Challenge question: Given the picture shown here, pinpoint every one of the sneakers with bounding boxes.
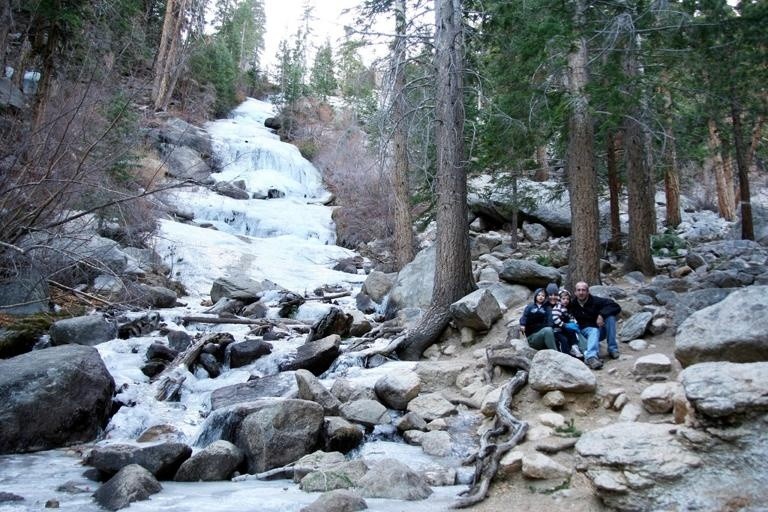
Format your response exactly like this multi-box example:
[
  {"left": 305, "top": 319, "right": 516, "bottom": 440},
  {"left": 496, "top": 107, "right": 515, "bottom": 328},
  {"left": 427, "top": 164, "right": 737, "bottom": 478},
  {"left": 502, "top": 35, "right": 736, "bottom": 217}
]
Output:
[
  {"left": 570, "top": 343, "right": 584, "bottom": 358},
  {"left": 609, "top": 351, "right": 620, "bottom": 359},
  {"left": 587, "top": 356, "right": 604, "bottom": 370}
]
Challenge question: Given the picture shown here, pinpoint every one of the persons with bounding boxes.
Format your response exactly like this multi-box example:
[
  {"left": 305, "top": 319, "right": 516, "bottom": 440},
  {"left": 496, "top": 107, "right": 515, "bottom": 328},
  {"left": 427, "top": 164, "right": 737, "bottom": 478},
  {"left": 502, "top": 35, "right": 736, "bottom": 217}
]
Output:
[{"left": 520, "top": 281, "right": 621, "bottom": 369}]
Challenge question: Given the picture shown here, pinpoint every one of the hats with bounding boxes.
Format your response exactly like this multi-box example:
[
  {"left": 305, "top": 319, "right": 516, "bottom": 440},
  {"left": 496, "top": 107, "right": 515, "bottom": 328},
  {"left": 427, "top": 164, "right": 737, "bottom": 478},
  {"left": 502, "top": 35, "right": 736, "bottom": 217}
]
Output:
[{"left": 546, "top": 283, "right": 559, "bottom": 294}]
